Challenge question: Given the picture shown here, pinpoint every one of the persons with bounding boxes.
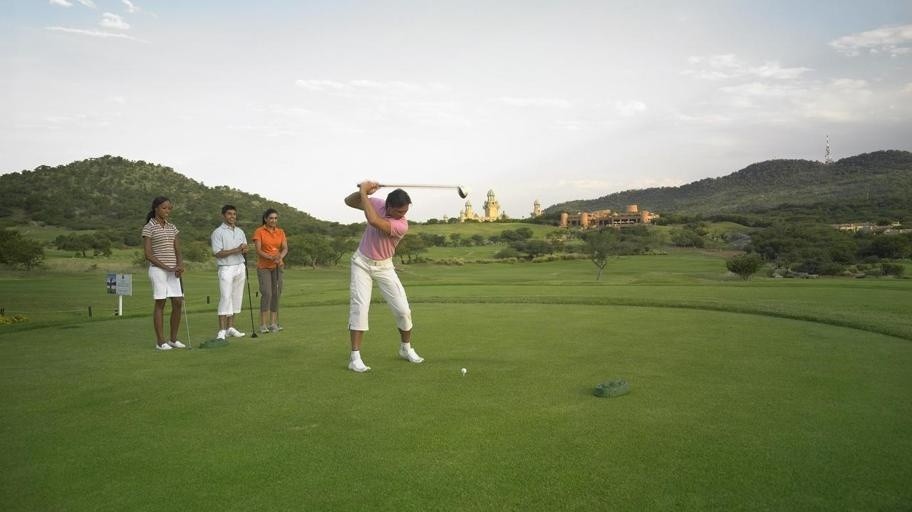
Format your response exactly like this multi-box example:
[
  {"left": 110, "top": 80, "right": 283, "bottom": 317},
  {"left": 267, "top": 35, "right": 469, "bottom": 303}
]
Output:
[
  {"left": 251, "top": 208, "right": 289, "bottom": 333},
  {"left": 140, "top": 196, "right": 186, "bottom": 351},
  {"left": 344, "top": 181, "right": 425, "bottom": 373},
  {"left": 210, "top": 204, "right": 250, "bottom": 341}
]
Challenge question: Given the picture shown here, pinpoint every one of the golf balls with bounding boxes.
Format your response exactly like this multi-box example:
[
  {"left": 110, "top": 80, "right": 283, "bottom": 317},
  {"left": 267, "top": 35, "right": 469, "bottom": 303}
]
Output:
[{"left": 461, "top": 368, "right": 466, "bottom": 374}]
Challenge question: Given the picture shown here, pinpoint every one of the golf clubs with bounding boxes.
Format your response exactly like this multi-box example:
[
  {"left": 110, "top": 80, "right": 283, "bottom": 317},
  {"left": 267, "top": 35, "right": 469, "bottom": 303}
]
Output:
[
  {"left": 180, "top": 274, "right": 192, "bottom": 349},
  {"left": 243, "top": 253, "right": 257, "bottom": 337},
  {"left": 357, "top": 184, "right": 467, "bottom": 199},
  {"left": 276, "top": 263, "right": 283, "bottom": 330}
]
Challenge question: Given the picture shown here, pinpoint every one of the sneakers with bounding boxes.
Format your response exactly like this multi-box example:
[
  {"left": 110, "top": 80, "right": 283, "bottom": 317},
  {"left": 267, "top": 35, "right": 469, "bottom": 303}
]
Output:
[
  {"left": 259, "top": 324, "right": 283, "bottom": 333},
  {"left": 348, "top": 359, "right": 371, "bottom": 373},
  {"left": 156, "top": 340, "right": 186, "bottom": 350},
  {"left": 399, "top": 348, "right": 424, "bottom": 363},
  {"left": 216, "top": 327, "right": 245, "bottom": 340}
]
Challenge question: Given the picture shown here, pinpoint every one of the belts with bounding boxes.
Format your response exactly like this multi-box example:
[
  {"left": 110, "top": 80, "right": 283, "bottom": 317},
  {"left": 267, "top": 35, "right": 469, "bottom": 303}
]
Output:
[{"left": 357, "top": 249, "right": 392, "bottom": 266}]
